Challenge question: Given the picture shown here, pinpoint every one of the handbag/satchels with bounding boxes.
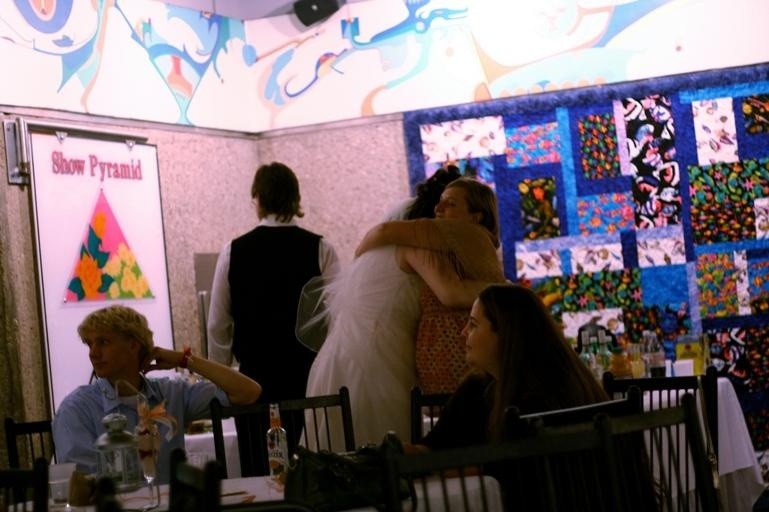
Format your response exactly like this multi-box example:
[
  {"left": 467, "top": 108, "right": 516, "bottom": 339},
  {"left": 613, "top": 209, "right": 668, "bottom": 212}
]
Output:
[{"left": 284, "top": 431, "right": 410, "bottom": 512}]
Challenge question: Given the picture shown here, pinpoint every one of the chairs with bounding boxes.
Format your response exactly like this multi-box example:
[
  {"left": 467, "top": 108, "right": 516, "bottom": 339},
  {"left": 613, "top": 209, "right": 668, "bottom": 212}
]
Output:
[
  {"left": 0, "top": 457, "right": 49, "bottom": 512},
  {"left": 605, "top": 407, "right": 703, "bottom": 512},
  {"left": 706, "top": 366, "right": 769, "bottom": 511},
  {"left": 409, "top": 385, "right": 455, "bottom": 445},
  {"left": 279, "top": 387, "right": 356, "bottom": 462},
  {"left": 209, "top": 397, "right": 271, "bottom": 480},
  {"left": 603, "top": 370, "right": 718, "bottom": 512},
  {"left": 381, "top": 427, "right": 595, "bottom": 512},
  {"left": 3, "top": 417, "right": 59, "bottom": 470}
]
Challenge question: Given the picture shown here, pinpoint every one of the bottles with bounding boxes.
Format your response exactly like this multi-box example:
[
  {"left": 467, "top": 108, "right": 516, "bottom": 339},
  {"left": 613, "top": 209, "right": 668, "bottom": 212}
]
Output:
[
  {"left": 578, "top": 331, "right": 596, "bottom": 369},
  {"left": 649, "top": 331, "right": 666, "bottom": 377},
  {"left": 95, "top": 414, "right": 141, "bottom": 493},
  {"left": 590, "top": 337, "right": 597, "bottom": 358},
  {"left": 267, "top": 403, "right": 290, "bottom": 481},
  {"left": 643, "top": 331, "right": 651, "bottom": 377},
  {"left": 596, "top": 330, "right": 611, "bottom": 369},
  {"left": 611, "top": 348, "right": 634, "bottom": 379}
]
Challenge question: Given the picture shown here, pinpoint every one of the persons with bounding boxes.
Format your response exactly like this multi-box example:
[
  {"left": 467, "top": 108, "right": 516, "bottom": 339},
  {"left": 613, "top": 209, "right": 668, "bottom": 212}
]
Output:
[
  {"left": 52, "top": 306, "right": 263, "bottom": 483},
  {"left": 353, "top": 179, "right": 503, "bottom": 394},
  {"left": 297, "top": 167, "right": 505, "bottom": 451},
  {"left": 206, "top": 162, "right": 339, "bottom": 478},
  {"left": 403, "top": 285, "right": 612, "bottom": 476}
]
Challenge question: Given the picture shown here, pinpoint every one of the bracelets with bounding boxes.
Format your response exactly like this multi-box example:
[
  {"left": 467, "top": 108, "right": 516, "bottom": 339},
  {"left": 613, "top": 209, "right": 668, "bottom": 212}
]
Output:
[{"left": 179, "top": 348, "right": 191, "bottom": 368}]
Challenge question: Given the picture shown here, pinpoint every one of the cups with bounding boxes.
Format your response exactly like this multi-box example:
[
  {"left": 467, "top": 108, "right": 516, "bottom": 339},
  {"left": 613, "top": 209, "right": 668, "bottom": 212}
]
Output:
[{"left": 49, "top": 462, "right": 78, "bottom": 505}]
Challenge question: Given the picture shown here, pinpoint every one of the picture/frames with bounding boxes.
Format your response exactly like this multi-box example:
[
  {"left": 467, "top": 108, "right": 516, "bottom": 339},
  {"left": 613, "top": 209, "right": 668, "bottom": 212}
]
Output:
[{"left": 28, "top": 127, "right": 178, "bottom": 424}]
